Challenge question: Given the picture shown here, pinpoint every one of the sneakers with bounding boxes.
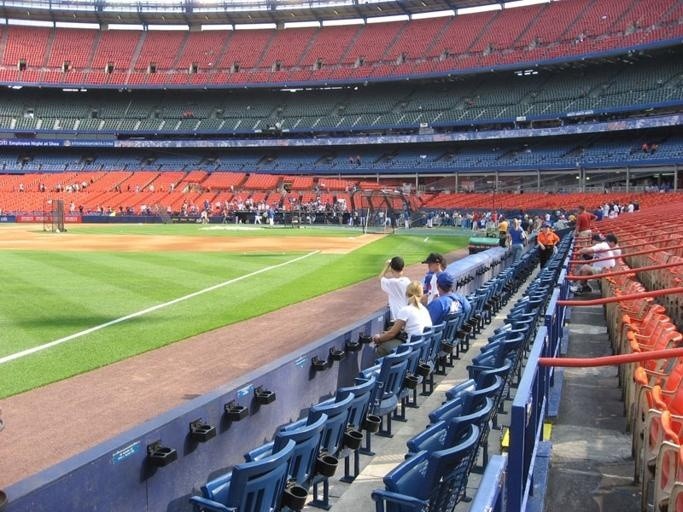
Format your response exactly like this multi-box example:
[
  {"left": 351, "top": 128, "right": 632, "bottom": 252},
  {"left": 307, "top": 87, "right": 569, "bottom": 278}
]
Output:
[{"left": 568, "top": 279, "right": 593, "bottom": 296}]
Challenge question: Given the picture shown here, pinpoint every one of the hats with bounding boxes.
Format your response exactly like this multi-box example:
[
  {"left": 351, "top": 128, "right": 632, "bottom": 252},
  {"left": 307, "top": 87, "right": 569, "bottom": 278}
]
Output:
[
  {"left": 436, "top": 271, "right": 455, "bottom": 289},
  {"left": 420, "top": 252, "right": 444, "bottom": 265},
  {"left": 604, "top": 232, "right": 618, "bottom": 242},
  {"left": 592, "top": 233, "right": 606, "bottom": 242},
  {"left": 389, "top": 256, "right": 404, "bottom": 268},
  {"left": 542, "top": 222, "right": 549, "bottom": 228}
]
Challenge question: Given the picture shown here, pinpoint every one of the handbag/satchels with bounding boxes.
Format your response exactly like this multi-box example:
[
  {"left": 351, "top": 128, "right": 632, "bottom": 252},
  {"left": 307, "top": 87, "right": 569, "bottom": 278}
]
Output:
[{"left": 519, "top": 227, "right": 526, "bottom": 241}]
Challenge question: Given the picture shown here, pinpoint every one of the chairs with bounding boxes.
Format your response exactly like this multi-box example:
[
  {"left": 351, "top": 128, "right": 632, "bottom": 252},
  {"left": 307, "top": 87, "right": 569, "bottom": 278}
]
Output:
[
  {"left": 190, "top": 393, "right": 502, "bottom": 510},
  {"left": 334, "top": 266, "right": 503, "bottom": 402},
  {"left": 502, "top": 2, "right": 681, "bottom": 512},
  {"left": 0, "top": 12, "right": 503, "bottom": 213}
]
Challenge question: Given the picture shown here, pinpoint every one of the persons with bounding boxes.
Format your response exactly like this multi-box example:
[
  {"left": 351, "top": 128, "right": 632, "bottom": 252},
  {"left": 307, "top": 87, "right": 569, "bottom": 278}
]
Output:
[{"left": 640, "top": 143, "right": 659, "bottom": 157}]
